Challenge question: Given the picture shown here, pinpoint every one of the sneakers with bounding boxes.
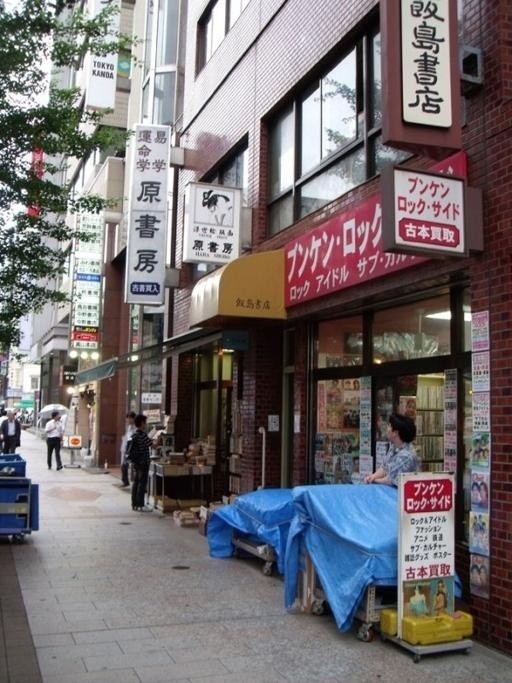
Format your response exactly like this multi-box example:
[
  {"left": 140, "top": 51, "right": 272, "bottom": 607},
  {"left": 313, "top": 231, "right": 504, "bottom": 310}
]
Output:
[{"left": 132, "top": 505, "right": 153, "bottom": 512}]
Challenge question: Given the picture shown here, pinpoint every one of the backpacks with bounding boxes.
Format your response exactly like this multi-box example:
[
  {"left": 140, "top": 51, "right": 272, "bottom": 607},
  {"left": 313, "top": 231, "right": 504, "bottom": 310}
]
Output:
[{"left": 123, "top": 436, "right": 140, "bottom": 463}]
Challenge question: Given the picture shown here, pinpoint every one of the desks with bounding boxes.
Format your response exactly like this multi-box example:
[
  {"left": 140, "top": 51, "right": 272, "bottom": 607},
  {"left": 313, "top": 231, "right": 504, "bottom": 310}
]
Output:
[{"left": 147, "top": 460, "right": 214, "bottom": 513}]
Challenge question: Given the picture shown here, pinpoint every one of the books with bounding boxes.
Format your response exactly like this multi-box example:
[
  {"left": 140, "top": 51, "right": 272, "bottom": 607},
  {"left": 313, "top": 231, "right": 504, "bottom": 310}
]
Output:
[
  {"left": 149, "top": 435, "right": 217, "bottom": 475},
  {"left": 149, "top": 488, "right": 238, "bottom": 528},
  {"left": 415, "top": 385, "right": 444, "bottom": 472}
]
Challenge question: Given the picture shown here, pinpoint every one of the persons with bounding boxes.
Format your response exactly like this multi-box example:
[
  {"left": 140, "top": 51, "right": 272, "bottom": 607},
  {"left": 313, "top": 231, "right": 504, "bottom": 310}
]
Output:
[
  {"left": 44, "top": 411, "right": 64, "bottom": 471},
  {"left": 14, "top": 409, "right": 33, "bottom": 426},
  {"left": 474, "top": 566, "right": 489, "bottom": 594},
  {"left": 325, "top": 378, "right": 362, "bottom": 484},
  {"left": 0, "top": 409, "right": 21, "bottom": 454},
  {"left": 404, "top": 398, "right": 417, "bottom": 418},
  {"left": 434, "top": 580, "right": 449, "bottom": 611},
  {"left": 470, "top": 434, "right": 489, "bottom": 546},
  {"left": 364, "top": 413, "right": 418, "bottom": 486},
  {"left": 470, "top": 564, "right": 479, "bottom": 592},
  {"left": 410, "top": 587, "right": 427, "bottom": 616},
  {"left": 124, "top": 415, "right": 160, "bottom": 512},
  {"left": 120, "top": 411, "right": 136, "bottom": 486},
  {"left": 0, "top": 411, "right": 8, "bottom": 451}
]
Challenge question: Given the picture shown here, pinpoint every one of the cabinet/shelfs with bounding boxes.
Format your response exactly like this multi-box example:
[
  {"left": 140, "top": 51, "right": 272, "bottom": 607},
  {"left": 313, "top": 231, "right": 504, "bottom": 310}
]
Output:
[{"left": 415, "top": 374, "right": 445, "bottom": 474}]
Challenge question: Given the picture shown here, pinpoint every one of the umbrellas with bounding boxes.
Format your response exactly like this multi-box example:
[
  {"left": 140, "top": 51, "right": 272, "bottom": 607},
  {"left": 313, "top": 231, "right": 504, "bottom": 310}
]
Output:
[{"left": 39, "top": 403, "right": 69, "bottom": 413}]
